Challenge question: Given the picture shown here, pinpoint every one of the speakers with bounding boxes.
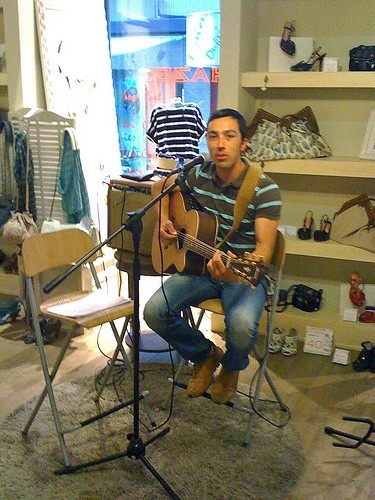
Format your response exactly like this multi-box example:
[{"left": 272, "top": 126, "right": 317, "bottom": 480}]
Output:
[{"left": 106, "top": 177, "right": 158, "bottom": 257}]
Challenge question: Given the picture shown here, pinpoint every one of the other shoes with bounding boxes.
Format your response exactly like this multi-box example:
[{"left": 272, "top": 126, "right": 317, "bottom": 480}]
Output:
[{"left": 352, "top": 341, "right": 375, "bottom": 373}]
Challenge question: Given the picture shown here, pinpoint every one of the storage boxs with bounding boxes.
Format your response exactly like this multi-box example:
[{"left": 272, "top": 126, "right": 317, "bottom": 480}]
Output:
[{"left": 107, "top": 177, "right": 157, "bottom": 256}]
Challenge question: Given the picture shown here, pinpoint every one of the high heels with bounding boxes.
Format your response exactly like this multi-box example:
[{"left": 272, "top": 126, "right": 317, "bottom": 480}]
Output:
[
  {"left": 298, "top": 211, "right": 314, "bottom": 240},
  {"left": 0, "top": 297, "right": 21, "bottom": 325},
  {"left": 268, "top": 327, "right": 299, "bottom": 356},
  {"left": 290, "top": 47, "right": 327, "bottom": 72},
  {"left": 348, "top": 271, "right": 365, "bottom": 306},
  {"left": 314, "top": 215, "right": 332, "bottom": 242},
  {"left": 281, "top": 18, "right": 295, "bottom": 56},
  {"left": 359, "top": 311, "right": 375, "bottom": 323},
  {"left": 24, "top": 317, "right": 61, "bottom": 346}
]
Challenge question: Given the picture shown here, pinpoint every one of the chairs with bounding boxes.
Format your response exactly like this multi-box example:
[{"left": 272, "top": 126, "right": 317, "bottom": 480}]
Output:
[
  {"left": 21, "top": 228, "right": 156, "bottom": 468},
  {"left": 161, "top": 226, "right": 286, "bottom": 446}
]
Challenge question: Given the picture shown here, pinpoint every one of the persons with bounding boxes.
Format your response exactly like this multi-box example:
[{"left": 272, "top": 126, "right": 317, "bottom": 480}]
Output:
[{"left": 143, "top": 109, "right": 281, "bottom": 405}]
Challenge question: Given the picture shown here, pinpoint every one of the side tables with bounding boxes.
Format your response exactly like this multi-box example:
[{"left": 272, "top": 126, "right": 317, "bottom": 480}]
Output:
[{"left": 113, "top": 251, "right": 188, "bottom": 352}]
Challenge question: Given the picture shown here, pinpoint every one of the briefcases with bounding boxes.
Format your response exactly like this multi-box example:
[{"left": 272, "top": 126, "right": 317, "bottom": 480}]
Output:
[{"left": 108, "top": 169, "right": 172, "bottom": 256}]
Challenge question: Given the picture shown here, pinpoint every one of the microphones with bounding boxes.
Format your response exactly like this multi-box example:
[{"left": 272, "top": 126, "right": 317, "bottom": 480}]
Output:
[{"left": 174, "top": 154, "right": 206, "bottom": 174}]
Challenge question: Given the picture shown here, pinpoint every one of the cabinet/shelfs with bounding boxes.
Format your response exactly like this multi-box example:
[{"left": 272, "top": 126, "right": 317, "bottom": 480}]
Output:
[{"left": 212, "top": 0, "right": 375, "bottom": 350}]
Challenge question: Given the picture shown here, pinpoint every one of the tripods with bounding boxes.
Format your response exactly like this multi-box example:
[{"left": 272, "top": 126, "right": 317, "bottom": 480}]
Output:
[{"left": 42, "top": 169, "right": 189, "bottom": 500}]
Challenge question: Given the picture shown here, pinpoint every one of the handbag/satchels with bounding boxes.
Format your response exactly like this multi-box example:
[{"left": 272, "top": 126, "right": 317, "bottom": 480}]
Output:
[
  {"left": 244, "top": 106, "right": 331, "bottom": 162},
  {"left": 265, "top": 285, "right": 323, "bottom": 313},
  {"left": 329, "top": 194, "right": 375, "bottom": 254},
  {"left": 3, "top": 213, "right": 38, "bottom": 244},
  {"left": 349, "top": 45, "right": 375, "bottom": 71}
]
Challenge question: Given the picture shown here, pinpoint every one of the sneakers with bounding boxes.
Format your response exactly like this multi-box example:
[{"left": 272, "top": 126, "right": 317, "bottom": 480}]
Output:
[
  {"left": 187, "top": 341, "right": 223, "bottom": 397},
  {"left": 211, "top": 366, "right": 238, "bottom": 405}
]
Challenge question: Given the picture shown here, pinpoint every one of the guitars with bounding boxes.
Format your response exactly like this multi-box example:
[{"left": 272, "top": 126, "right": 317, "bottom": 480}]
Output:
[{"left": 151, "top": 191, "right": 269, "bottom": 290}]
